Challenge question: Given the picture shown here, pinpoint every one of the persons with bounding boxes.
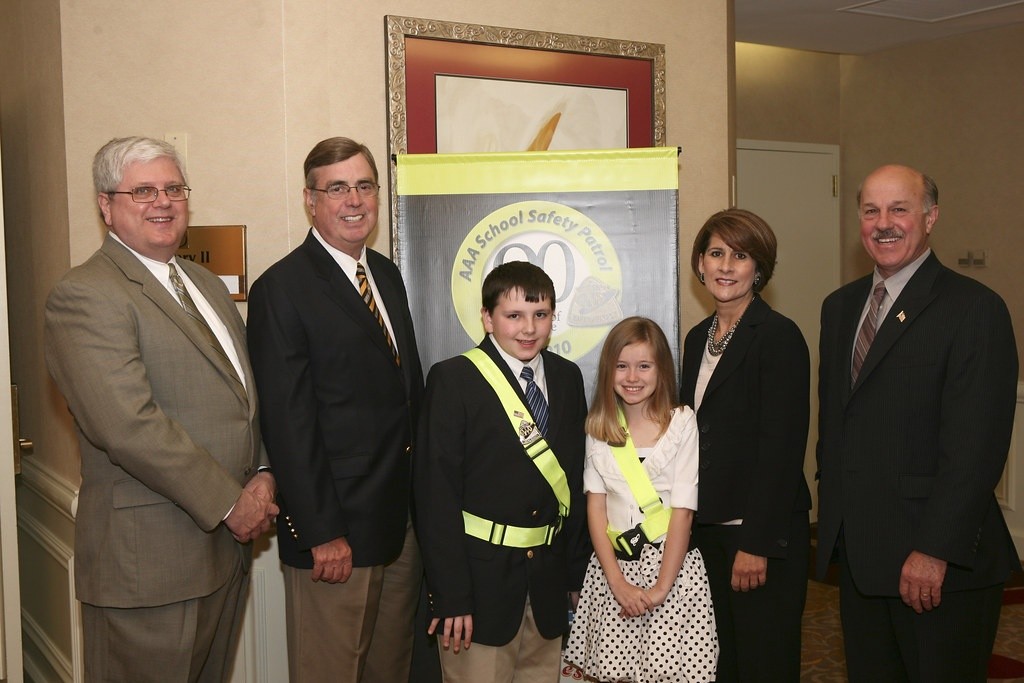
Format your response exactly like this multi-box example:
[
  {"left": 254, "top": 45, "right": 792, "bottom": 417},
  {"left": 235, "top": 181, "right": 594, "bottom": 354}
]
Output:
[
  {"left": 817, "top": 163, "right": 1022, "bottom": 683},
  {"left": 248, "top": 138, "right": 424, "bottom": 683},
  {"left": 680, "top": 206, "right": 814, "bottom": 576},
  {"left": 415, "top": 259, "right": 589, "bottom": 683},
  {"left": 564, "top": 316, "right": 722, "bottom": 683},
  {"left": 44, "top": 133, "right": 277, "bottom": 683}
]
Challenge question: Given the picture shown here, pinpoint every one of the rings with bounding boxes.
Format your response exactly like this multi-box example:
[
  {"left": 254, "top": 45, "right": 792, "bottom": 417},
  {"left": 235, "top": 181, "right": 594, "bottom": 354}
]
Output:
[{"left": 921, "top": 594, "right": 930, "bottom": 601}]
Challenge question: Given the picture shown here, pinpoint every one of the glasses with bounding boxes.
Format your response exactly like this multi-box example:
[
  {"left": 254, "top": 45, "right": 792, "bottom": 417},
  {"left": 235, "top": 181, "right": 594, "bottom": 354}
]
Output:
[
  {"left": 108, "top": 184, "right": 192, "bottom": 203},
  {"left": 310, "top": 183, "right": 380, "bottom": 200}
]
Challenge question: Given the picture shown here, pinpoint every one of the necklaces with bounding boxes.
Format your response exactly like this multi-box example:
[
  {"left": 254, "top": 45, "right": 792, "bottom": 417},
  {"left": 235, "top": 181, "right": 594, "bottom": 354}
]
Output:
[{"left": 710, "top": 310, "right": 745, "bottom": 355}]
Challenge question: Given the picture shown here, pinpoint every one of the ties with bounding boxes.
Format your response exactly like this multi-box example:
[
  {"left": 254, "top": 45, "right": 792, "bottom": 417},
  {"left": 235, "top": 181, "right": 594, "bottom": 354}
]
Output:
[
  {"left": 356, "top": 263, "right": 401, "bottom": 370},
  {"left": 167, "top": 263, "right": 249, "bottom": 405},
  {"left": 520, "top": 367, "right": 549, "bottom": 439},
  {"left": 850, "top": 281, "right": 887, "bottom": 391}
]
